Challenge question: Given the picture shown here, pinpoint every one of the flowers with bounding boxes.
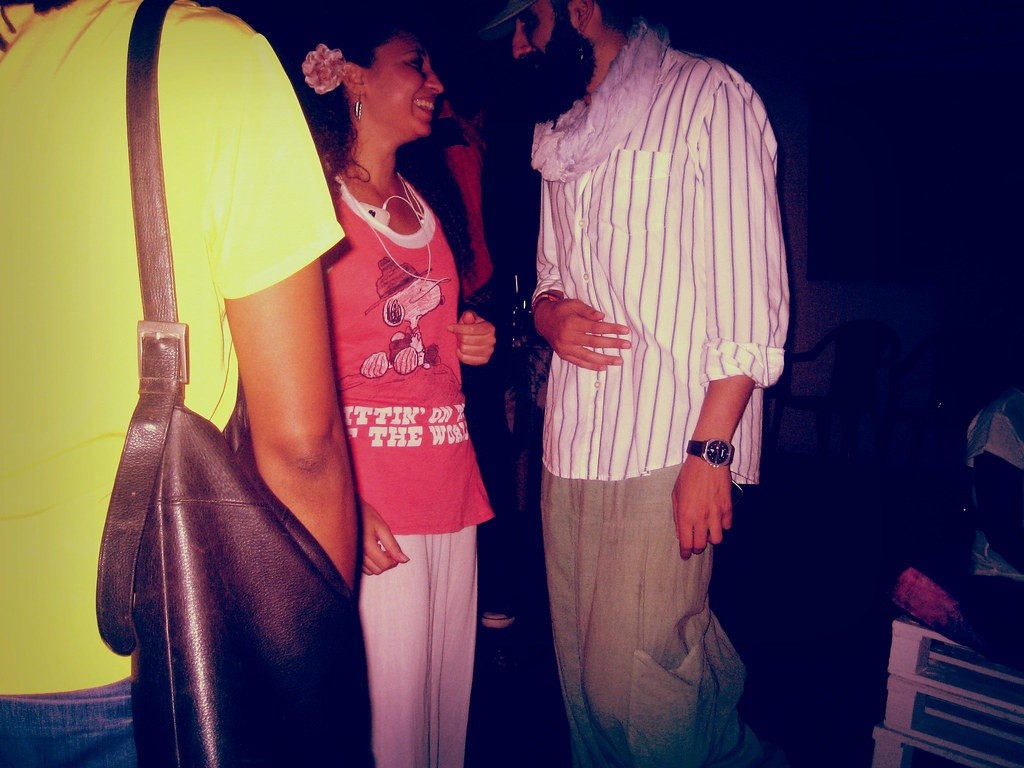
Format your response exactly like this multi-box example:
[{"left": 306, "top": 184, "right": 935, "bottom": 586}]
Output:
[{"left": 300, "top": 44, "right": 345, "bottom": 95}]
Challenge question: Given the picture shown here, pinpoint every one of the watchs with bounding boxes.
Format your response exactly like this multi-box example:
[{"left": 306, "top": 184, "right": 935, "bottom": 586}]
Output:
[{"left": 685, "top": 437, "right": 735, "bottom": 468}]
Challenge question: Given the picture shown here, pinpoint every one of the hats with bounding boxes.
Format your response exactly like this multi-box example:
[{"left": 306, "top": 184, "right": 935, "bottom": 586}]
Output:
[{"left": 478, "top": 0, "right": 539, "bottom": 41}]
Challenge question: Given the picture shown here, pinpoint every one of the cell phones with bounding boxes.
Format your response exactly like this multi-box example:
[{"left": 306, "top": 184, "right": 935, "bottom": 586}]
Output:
[{"left": 357, "top": 202, "right": 390, "bottom": 228}]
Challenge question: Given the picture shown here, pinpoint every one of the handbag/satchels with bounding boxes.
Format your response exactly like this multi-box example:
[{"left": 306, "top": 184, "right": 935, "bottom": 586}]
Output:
[{"left": 97, "top": 318, "right": 370, "bottom": 768}]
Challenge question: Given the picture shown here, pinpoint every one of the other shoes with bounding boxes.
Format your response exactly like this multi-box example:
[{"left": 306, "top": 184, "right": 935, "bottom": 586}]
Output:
[{"left": 482, "top": 574, "right": 523, "bottom": 628}]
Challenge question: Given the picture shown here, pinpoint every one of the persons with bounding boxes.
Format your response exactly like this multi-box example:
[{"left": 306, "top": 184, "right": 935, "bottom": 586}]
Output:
[
  {"left": 483, "top": 0, "right": 790, "bottom": 768},
  {"left": 0, "top": 0, "right": 497, "bottom": 768},
  {"left": 965, "top": 384, "right": 1024, "bottom": 655}
]
